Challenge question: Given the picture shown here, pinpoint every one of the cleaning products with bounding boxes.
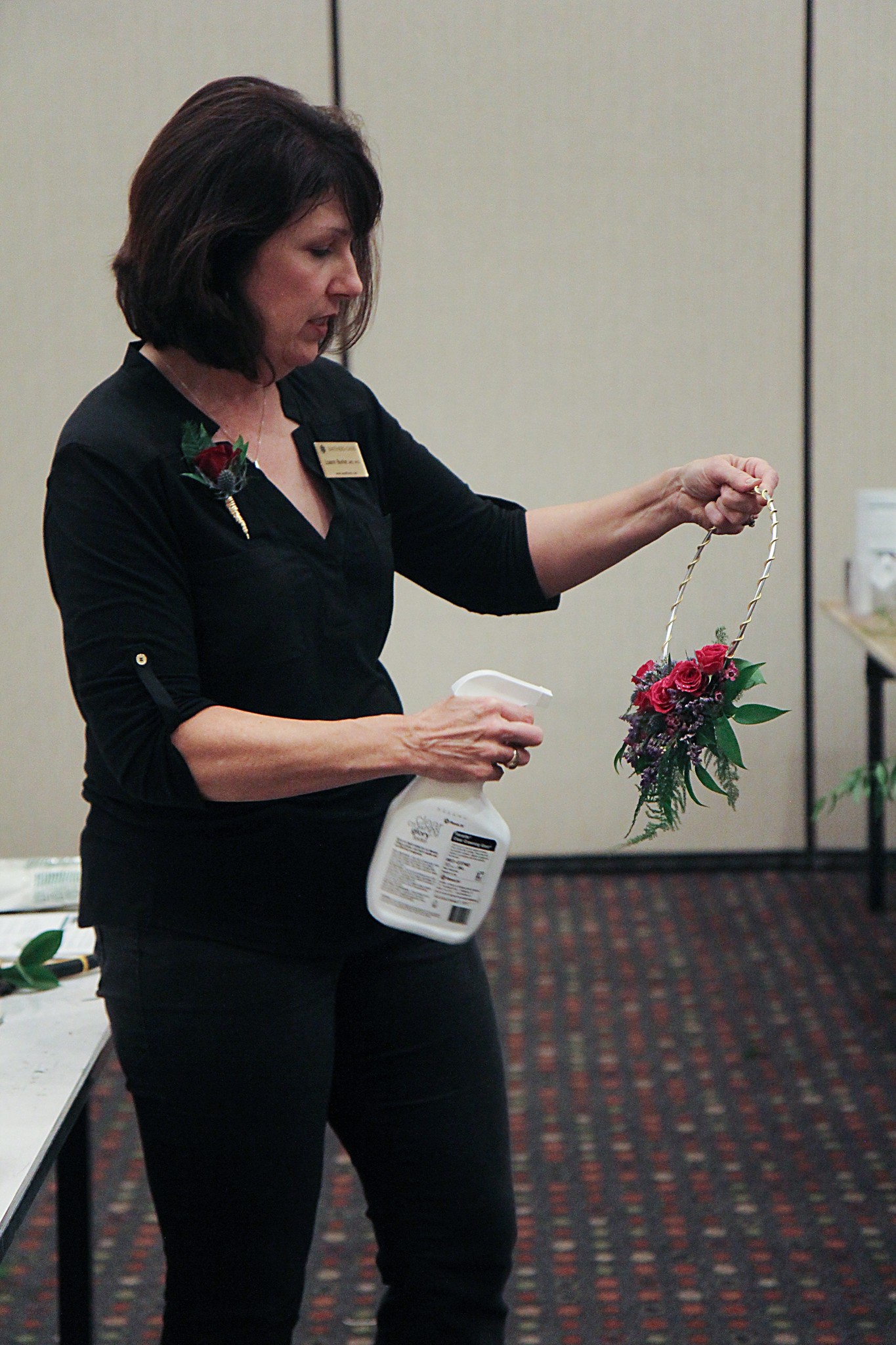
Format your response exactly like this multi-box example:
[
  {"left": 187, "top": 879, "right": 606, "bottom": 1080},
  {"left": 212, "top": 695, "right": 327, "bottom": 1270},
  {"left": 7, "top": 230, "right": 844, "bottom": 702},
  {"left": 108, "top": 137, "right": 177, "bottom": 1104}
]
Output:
[{"left": 367, "top": 671, "right": 553, "bottom": 946}]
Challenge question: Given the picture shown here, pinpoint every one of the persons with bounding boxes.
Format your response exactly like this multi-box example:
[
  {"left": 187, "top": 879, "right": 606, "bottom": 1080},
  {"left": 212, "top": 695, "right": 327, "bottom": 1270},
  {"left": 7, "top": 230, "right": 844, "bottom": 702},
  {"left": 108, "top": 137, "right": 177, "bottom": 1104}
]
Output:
[{"left": 42, "top": 74, "right": 781, "bottom": 1345}]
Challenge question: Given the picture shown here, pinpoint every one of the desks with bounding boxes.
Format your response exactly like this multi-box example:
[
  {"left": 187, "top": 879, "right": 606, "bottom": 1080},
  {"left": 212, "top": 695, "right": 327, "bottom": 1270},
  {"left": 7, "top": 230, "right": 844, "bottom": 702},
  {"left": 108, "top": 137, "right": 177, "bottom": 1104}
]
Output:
[
  {"left": 815, "top": 597, "right": 896, "bottom": 916},
  {"left": 0, "top": 856, "right": 116, "bottom": 1345}
]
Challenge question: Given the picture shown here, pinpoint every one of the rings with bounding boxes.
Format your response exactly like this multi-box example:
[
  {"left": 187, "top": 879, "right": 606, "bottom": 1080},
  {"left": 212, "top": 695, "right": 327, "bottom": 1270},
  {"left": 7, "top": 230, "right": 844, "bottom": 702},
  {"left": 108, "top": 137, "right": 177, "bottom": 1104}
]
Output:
[{"left": 506, "top": 749, "right": 519, "bottom": 769}]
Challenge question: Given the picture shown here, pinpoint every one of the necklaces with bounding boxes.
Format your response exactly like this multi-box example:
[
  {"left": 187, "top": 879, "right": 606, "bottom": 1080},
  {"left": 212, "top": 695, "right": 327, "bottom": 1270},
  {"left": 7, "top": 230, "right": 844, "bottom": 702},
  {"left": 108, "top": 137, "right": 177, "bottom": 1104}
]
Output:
[{"left": 151, "top": 348, "right": 266, "bottom": 470}]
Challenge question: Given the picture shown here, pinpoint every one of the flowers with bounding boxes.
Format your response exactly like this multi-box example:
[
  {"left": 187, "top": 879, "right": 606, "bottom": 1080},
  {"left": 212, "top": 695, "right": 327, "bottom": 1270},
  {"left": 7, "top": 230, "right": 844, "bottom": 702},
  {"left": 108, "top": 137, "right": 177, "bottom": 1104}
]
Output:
[{"left": 611, "top": 613, "right": 789, "bottom": 850}]
